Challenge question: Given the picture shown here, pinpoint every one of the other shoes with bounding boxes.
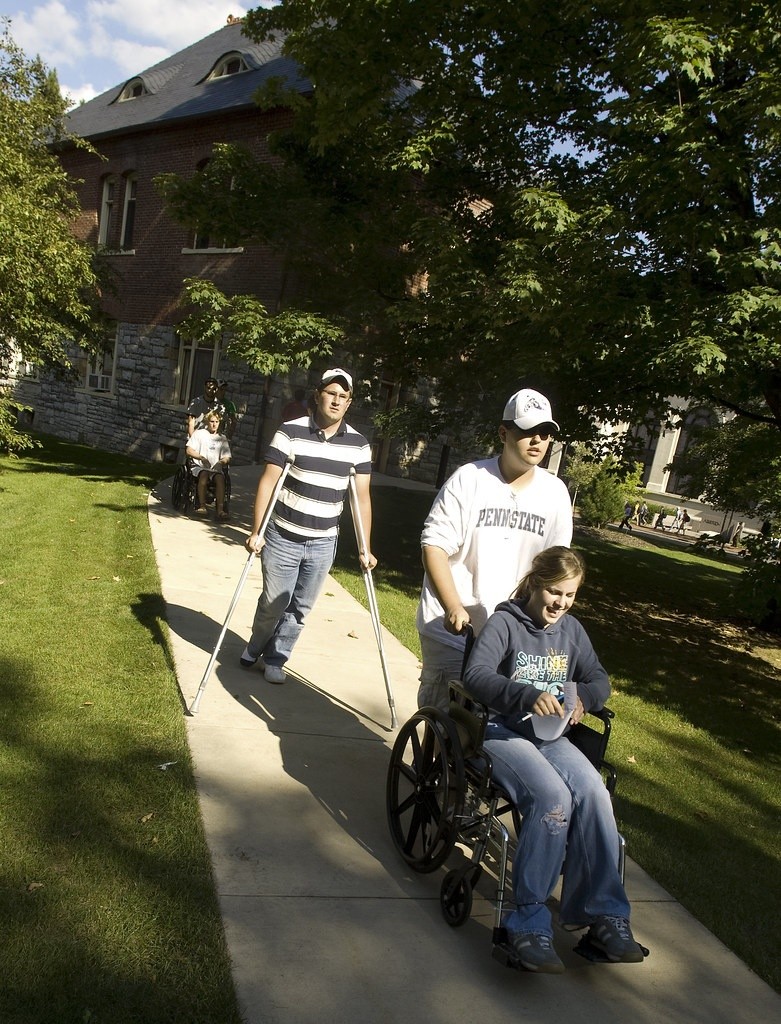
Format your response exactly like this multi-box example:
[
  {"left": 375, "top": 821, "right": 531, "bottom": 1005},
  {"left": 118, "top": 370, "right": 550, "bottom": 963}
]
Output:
[
  {"left": 197, "top": 506, "right": 207, "bottom": 515},
  {"left": 217, "top": 509, "right": 229, "bottom": 519},
  {"left": 439, "top": 801, "right": 482, "bottom": 843}
]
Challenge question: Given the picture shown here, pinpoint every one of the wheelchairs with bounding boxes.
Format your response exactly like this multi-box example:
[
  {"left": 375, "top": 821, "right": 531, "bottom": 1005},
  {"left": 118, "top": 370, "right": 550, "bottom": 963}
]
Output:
[
  {"left": 171, "top": 439, "right": 231, "bottom": 520},
  {"left": 384, "top": 621, "right": 651, "bottom": 972}
]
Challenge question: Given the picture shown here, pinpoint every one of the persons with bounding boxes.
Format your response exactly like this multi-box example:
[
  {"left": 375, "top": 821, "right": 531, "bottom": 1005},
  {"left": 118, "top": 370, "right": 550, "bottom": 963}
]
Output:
[
  {"left": 183, "top": 377, "right": 237, "bottom": 521},
  {"left": 618, "top": 499, "right": 689, "bottom": 537},
  {"left": 730, "top": 522, "right": 746, "bottom": 548},
  {"left": 461, "top": 544, "right": 646, "bottom": 975},
  {"left": 280, "top": 390, "right": 309, "bottom": 425},
  {"left": 412, "top": 390, "right": 574, "bottom": 756},
  {"left": 239, "top": 367, "right": 379, "bottom": 682}
]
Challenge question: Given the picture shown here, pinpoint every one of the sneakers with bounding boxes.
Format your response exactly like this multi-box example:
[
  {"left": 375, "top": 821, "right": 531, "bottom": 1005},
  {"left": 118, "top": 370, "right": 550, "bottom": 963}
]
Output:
[
  {"left": 507, "top": 928, "right": 563, "bottom": 974},
  {"left": 589, "top": 914, "right": 643, "bottom": 963},
  {"left": 264, "top": 656, "right": 286, "bottom": 684}
]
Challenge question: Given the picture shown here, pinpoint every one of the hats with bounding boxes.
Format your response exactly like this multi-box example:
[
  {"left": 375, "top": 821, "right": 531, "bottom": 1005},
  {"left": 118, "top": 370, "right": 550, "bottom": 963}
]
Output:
[
  {"left": 216, "top": 378, "right": 228, "bottom": 388},
  {"left": 319, "top": 370, "right": 354, "bottom": 394},
  {"left": 503, "top": 389, "right": 561, "bottom": 434}
]
